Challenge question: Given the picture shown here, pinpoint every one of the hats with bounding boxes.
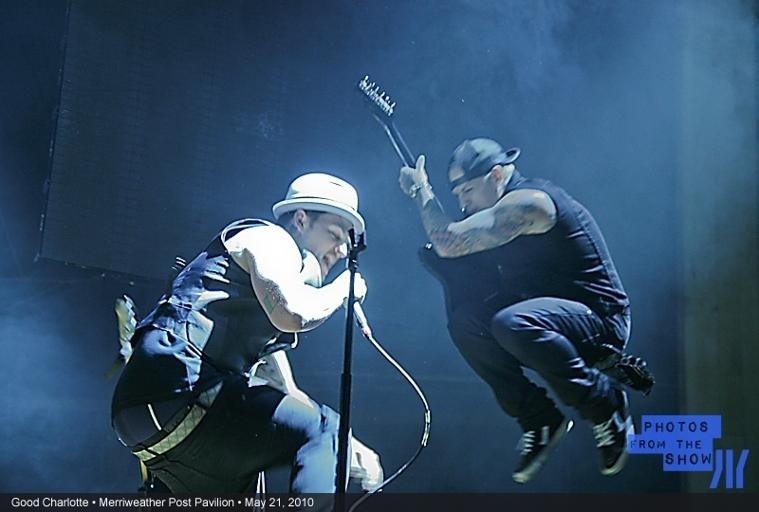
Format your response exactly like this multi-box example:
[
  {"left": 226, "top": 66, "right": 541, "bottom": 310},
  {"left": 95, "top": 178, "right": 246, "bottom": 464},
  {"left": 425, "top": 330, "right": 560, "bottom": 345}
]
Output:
[
  {"left": 272, "top": 172, "right": 366, "bottom": 239},
  {"left": 446, "top": 137, "right": 521, "bottom": 193}
]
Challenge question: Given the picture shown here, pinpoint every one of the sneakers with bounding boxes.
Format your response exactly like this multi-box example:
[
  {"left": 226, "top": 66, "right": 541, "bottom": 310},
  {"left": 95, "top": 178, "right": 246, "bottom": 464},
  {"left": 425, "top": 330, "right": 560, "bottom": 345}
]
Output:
[
  {"left": 511, "top": 406, "right": 575, "bottom": 485},
  {"left": 590, "top": 389, "right": 637, "bottom": 476}
]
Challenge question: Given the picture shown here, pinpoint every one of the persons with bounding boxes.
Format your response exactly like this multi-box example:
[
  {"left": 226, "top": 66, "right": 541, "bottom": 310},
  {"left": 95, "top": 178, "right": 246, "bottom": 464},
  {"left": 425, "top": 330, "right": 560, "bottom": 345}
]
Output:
[
  {"left": 396, "top": 137, "right": 637, "bottom": 484},
  {"left": 107, "top": 173, "right": 386, "bottom": 501}
]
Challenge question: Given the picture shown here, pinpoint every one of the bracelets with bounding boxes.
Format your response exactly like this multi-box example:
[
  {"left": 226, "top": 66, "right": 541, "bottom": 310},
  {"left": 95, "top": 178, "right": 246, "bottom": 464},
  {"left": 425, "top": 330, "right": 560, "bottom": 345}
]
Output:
[{"left": 407, "top": 181, "right": 434, "bottom": 203}]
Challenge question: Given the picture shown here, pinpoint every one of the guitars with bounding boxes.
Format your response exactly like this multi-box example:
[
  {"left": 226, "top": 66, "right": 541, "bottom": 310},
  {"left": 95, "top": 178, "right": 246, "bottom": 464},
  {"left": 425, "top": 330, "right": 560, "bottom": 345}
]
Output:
[{"left": 357, "top": 75, "right": 503, "bottom": 321}]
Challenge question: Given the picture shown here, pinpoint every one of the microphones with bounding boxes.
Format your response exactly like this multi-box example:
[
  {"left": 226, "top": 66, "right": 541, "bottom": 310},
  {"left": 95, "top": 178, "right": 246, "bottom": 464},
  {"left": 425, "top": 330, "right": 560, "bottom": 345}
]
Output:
[{"left": 339, "top": 290, "right": 374, "bottom": 339}]
